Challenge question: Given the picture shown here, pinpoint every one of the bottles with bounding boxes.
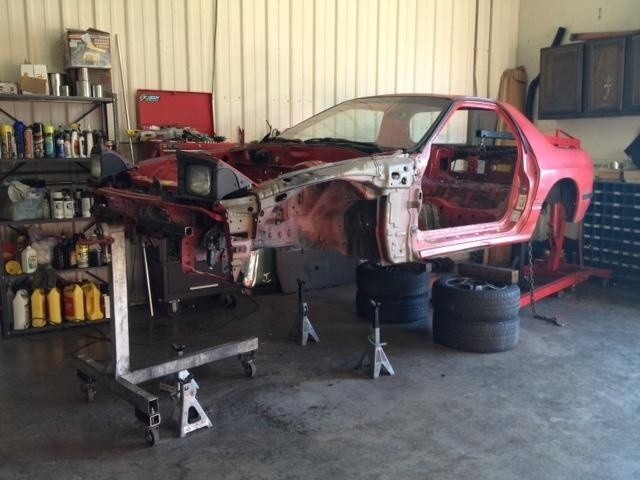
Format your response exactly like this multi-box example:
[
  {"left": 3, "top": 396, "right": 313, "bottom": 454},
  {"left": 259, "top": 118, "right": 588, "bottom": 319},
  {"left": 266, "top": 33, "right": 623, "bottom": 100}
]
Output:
[
  {"left": 22, "top": 242, "right": 37, "bottom": 273},
  {"left": 58, "top": 133, "right": 65, "bottom": 158},
  {"left": 44, "top": 125, "right": 56, "bottom": 157},
  {"left": 34, "top": 123, "right": 45, "bottom": 158},
  {"left": 25, "top": 128, "right": 34, "bottom": 158},
  {"left": 65, "top": 131, "right": 71, "bottom": 158},
  {"left": 75, "top": 188, "right": 82, "bottom": 216},
  {"left": 82, "top": 190, "right": 92, "bottom": 217},
  {"left": 76, "top": 239, "right": 89, "bottom": 268},
  {"left": 2, "top": 124, "right": 12, "bottom": 159},
  {"left": 90, "top": 233, "right": 102, "bottom": 267},
  {"left": 64, "top": 194, "right": 73, "bottom": 218},
  {"left": 52, "top": 192, "right": 64, "bottom": 219},
  {"left": 14, "top": 121, "right": 25, "bottom": 159}
]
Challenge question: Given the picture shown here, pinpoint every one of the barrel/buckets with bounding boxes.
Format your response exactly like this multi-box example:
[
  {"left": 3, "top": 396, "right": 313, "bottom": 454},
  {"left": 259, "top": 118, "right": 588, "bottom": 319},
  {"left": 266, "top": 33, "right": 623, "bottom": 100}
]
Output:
[
  {"left": 31, "top": 288, "right": 47, "bottom": 327},
  {"left": 64, "top": 284, "right": 84, "bottom": 322},
  {"left": 81, "top": 283, "right": 104, "bottom": 320},
  {"left": 47, "top": 287, "right": 62, "bottom": 325},
  {"left": 13, "top": 289, "right": 30, "bottom": 330}
]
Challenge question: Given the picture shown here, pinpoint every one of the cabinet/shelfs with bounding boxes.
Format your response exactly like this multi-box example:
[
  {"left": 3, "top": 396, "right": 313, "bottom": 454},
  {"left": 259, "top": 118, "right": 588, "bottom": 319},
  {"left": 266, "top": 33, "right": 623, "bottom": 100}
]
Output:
[
  {"left": 629, "top": 35, "right": 640, "bottom": 110},
  {"left": 539, "top": 43, "right": 583, "bottom": 115},
  {"left": 0, "top": 90, "right": 120, "bottom": 341},
  {"left": 586, "top": 38, "right": 623, "bottom": 112}
]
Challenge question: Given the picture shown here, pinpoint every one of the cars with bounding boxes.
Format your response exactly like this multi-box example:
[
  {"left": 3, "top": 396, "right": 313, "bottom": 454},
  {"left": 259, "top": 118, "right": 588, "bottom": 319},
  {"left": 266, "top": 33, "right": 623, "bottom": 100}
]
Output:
[{"left": 86, "top": 92, "right": 595, "bottom": 290}]
